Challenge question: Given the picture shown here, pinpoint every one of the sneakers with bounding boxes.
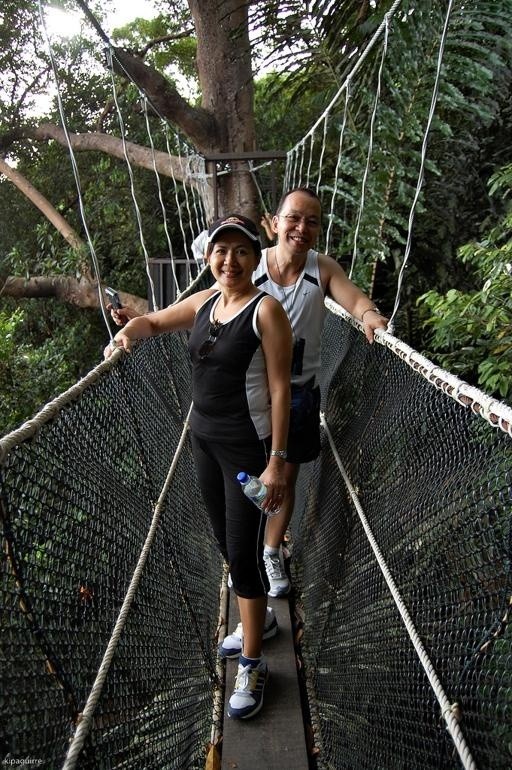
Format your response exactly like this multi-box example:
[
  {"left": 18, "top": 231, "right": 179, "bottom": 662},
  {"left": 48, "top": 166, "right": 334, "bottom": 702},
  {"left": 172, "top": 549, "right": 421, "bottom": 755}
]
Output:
[
  {"left": 228, "top": 662, "right": 268, "bottom": 720},
  {"left": 216, "top": 609, "right": 276, "bottom": 659},
  {"left": 263, "top": 553, "right": 292, "bottom": 599}
]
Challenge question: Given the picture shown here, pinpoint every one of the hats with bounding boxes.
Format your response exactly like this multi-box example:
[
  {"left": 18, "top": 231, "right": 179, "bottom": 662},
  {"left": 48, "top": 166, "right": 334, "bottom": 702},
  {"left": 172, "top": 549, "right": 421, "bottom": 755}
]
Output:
[{"left": 208, "top": 215, "right": 263, "bottom": 247}]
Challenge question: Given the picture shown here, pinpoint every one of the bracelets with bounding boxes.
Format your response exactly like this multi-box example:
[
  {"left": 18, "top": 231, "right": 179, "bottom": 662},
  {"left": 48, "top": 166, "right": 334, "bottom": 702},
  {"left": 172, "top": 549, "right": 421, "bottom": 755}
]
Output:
[{"left": 359, "top": 307, "right": 384, "bottom": 322}]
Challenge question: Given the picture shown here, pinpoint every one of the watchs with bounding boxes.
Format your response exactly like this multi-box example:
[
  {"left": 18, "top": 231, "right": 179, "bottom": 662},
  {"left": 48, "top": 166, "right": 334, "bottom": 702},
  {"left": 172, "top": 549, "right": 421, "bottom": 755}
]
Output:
[{"left": 270, "top": 448, "right": 289, "bottom": 462}]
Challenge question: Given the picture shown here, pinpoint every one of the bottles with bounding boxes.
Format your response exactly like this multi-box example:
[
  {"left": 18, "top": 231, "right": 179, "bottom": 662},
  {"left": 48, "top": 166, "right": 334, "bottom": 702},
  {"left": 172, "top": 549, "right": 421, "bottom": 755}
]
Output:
[{"left": 237, "top": 471, "right": 285, "bottom": 517}]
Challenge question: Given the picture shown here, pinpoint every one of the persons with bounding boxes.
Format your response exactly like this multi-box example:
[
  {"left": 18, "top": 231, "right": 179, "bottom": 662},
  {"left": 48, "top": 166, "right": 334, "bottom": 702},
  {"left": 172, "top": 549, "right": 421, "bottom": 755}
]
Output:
[
  {"left": 103, "top": 186, "right": 391, "bottom": 606},
  {"left": 99, "top": 213, "right": 296, "bottom": 727},
  {"left": 190, "top": 215, "right": 219, "bottom": 272},
  {"left": 258, "top": 210, "right": 279, "bottom": 249}
]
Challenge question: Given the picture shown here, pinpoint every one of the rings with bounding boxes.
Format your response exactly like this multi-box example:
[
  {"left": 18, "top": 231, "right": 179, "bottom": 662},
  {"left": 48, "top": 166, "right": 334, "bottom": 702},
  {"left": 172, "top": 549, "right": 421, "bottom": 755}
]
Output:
[{"left": 277, "top": 493, "right": 283, "bottom": 498}]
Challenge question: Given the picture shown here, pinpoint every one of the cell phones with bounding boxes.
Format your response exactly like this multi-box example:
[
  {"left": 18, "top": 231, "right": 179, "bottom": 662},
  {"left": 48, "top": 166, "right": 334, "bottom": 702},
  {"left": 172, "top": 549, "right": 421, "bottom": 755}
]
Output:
[{"left": 105, "top": 286, "right": 126, "bottom": 325}]
